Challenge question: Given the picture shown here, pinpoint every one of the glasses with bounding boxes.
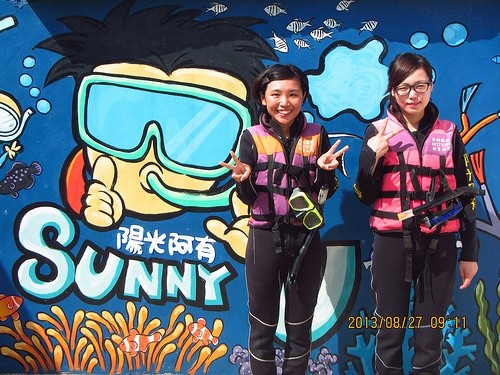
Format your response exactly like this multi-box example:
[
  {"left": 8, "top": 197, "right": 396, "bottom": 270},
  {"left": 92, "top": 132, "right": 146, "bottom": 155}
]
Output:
[
  {"left": 392, "top": 79, "right": 432, "bottom": 96},
  {"left": 290, "top": 189, "right": 323, "bottom": 230}
]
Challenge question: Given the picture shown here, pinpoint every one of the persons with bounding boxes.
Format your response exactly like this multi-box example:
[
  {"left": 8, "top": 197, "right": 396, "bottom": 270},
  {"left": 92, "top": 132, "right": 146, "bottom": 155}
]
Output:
[
  {"left": 219, "top": 62, "right": 349, "bottom": 374},
  {"left": 353, "top": 51, "right": 479, "bottom": 374}
]
